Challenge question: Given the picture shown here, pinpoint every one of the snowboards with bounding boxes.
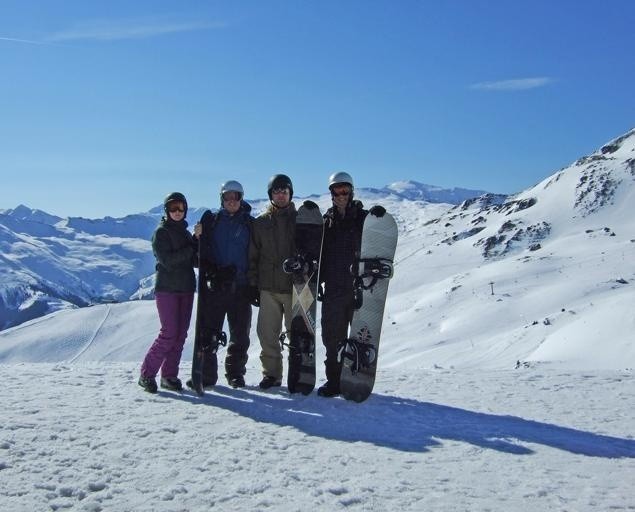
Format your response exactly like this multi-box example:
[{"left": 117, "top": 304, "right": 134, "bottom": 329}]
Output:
[
  {"left": 340, "top": 213, "right": 398, "bottom": 403},
  {"left": 288, "top": 205, "right": 325, "bottom": 396},
  {"left": 192, "top": 210, "right": 211, "bottom": 396}
]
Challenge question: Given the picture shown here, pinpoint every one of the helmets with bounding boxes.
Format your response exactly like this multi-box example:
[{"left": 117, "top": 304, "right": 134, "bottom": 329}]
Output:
[
  {"left": 219, "top": 180, "right": 244, "bottom": 196},
  {"left": 328, "top": 171, "right": 353, "bottom": 189},
  {"left": 164, "top": 192, "right": 187, "bottom": 206},
  {"left": 267, "top": 174, "right": 293, "bottom": 191}
]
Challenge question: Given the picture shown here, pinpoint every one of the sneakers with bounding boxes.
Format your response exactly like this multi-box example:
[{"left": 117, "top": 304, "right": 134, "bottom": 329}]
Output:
[{"left": 138, "top": 371, "right": 340, "bottom": 398}]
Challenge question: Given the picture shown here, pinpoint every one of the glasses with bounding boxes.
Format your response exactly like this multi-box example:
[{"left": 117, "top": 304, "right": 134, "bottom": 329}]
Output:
[
  {"left": 222, "top": 192, "right": 242, "bottom": 201},
  {"left": 166, "top": 201, "right": 187, "bottom": 212},
  {"left": 331, "top": 186, "right": 352, "bottom": 196},
  {"left": 271, "top": 185, "right": 292, "bottom": 194}
]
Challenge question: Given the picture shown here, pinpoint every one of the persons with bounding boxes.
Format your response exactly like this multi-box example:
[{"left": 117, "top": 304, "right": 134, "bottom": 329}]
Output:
[
  {"left": 315, "top": 170, "right": 387, "bottom": 398},
  {"left": 136, "top": 191, "right": 199, "bottom": 395},
  {"left": 245, "top": 173, "right": 320, "bottom": 389},
  {"left": 186, "top": 180, "right": 258, "bottom": 389}
]
